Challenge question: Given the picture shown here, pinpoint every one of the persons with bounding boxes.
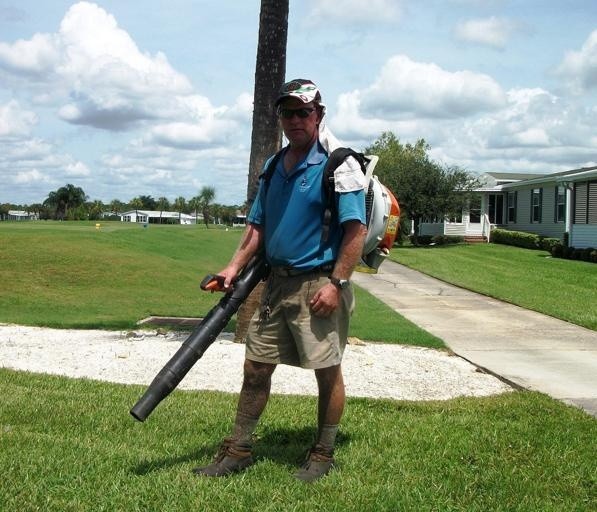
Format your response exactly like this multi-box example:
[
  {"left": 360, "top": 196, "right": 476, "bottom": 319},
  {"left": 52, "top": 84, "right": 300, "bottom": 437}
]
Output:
[{"left": 192, "top": 78, "right": 371, "bottom": 486}]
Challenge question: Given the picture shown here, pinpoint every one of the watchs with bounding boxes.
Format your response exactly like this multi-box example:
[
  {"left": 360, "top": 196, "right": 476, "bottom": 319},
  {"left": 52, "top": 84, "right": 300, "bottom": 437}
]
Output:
[{"left": 331, "top": 276, "right": 348, "bottom": 290}]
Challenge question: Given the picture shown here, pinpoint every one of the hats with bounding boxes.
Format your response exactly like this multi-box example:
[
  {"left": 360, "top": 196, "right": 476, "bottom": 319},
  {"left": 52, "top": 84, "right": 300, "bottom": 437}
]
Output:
[{"left": 274, "top": 79, "right": 326, "bottom": 108}]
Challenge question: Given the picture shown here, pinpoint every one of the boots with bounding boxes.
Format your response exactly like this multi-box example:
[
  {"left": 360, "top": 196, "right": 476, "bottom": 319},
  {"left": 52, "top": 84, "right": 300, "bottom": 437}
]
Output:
[
  {"left": 192, "top": 438, "right": 254, "bottom": 477},
  {"left": 290, "top": 447, "right": 336, "bottom": 483}
]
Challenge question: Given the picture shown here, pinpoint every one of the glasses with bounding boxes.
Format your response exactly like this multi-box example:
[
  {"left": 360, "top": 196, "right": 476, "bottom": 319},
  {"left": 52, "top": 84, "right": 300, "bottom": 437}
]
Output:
[{"left": 279, "top": 107, "right": 316, "bottom": 118}]
